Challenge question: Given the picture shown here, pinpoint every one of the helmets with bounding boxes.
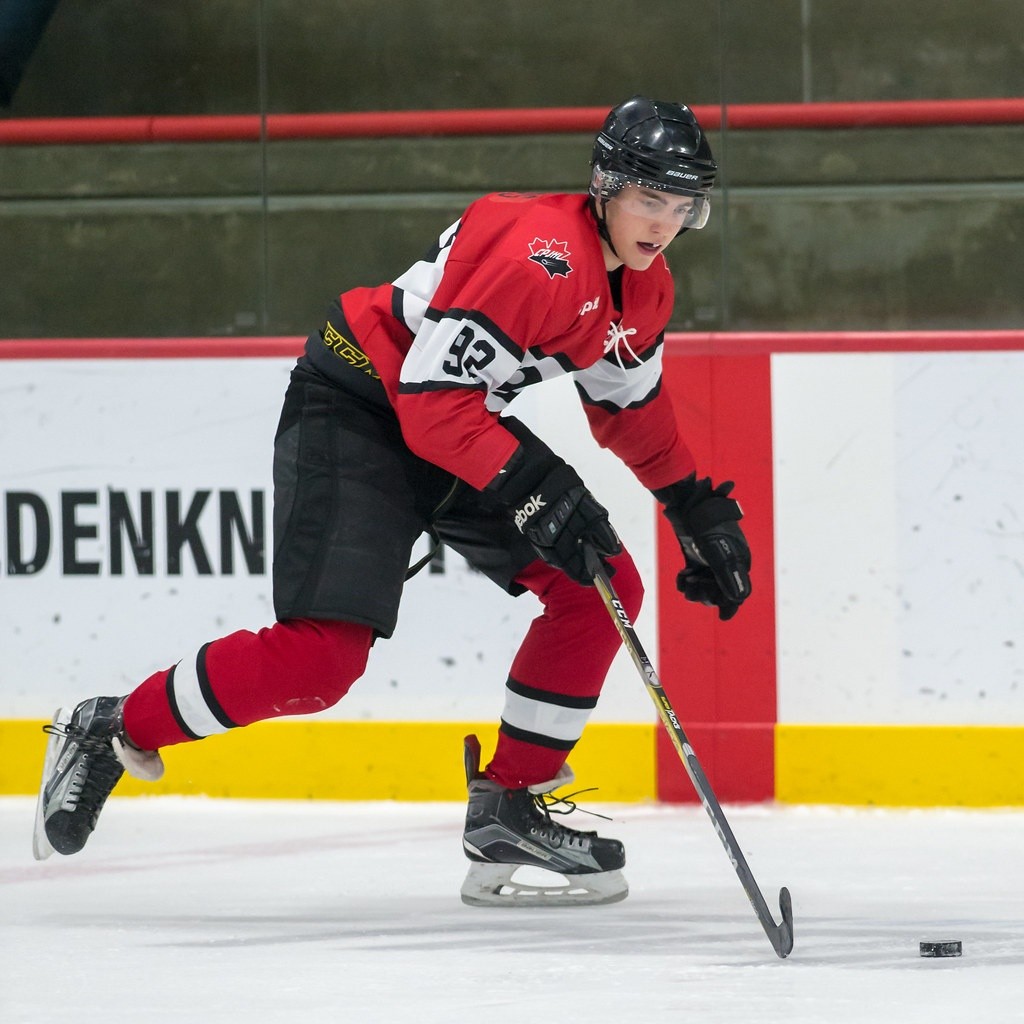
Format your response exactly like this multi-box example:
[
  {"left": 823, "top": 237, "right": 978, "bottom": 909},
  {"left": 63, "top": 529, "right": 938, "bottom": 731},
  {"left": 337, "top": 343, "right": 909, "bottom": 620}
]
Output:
[{"left": 589, "top": 95, "right": 718, "bottom": 224}]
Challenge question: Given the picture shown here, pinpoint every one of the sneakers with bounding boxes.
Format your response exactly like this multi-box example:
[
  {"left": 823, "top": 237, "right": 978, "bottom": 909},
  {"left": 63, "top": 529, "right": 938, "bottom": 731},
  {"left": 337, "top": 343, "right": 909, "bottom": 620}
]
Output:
[
  {"left": 461, "top": 734, "right": 630, "bottom": 907},
  {"left": 33, "top": 694, "right": 165, "bottom": 860}
]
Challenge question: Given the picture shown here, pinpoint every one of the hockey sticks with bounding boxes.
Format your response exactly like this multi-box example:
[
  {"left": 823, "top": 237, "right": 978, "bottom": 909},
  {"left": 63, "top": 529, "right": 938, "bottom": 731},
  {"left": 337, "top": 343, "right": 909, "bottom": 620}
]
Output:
[{"left": 581, "top": 544, "right": 795, "bottom": 958}]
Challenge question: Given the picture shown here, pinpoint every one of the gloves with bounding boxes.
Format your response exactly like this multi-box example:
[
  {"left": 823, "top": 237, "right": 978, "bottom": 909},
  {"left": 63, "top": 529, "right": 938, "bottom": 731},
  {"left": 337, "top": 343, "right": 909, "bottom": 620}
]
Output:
[
  {"left": 662, "top": 476, "right": 753, "bottom": 621},
  {"left": 490, "top": 449, "right": 621, "bottom": 586}
]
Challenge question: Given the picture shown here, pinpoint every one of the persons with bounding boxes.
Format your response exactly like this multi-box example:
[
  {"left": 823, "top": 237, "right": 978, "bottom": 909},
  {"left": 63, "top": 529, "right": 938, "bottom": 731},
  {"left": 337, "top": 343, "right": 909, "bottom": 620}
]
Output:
[{"left": 32, "top": 97, "right": 751, "bottom": 907}]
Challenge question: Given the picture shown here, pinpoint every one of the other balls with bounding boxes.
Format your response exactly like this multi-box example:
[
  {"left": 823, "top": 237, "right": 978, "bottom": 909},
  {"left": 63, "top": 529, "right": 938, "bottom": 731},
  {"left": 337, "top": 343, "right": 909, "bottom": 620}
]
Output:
[{"left": 919, "top": 939, "right": 963, "bottom": 958}]
